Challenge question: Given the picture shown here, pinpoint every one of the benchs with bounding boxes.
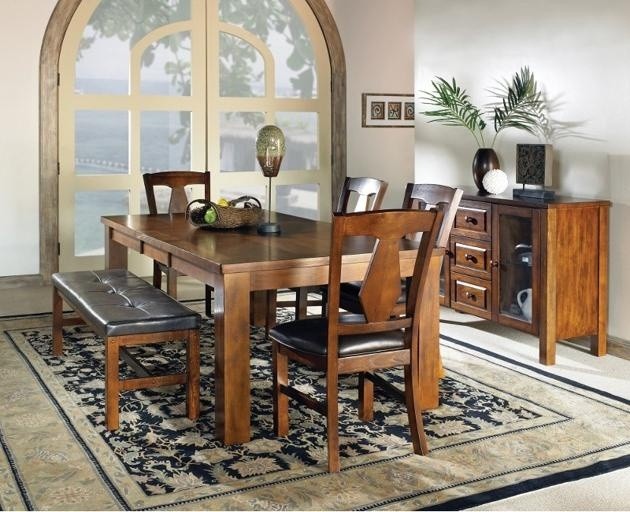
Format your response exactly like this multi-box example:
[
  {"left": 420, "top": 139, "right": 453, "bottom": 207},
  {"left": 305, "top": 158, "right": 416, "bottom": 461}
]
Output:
[{"left": 51, "top": 268, "right": 201, "bottom": 430}]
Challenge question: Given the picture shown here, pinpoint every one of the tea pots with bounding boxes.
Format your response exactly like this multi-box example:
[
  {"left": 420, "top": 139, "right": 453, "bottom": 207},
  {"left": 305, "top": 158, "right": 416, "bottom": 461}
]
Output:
[{"left": 517, "top": 288, "right": 532, "bottom": 322}]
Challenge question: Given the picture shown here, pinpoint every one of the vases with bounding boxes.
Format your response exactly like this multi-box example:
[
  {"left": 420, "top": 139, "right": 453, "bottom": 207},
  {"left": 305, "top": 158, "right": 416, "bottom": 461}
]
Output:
[{"left": 473, "top": 148, "right": 499, "bottom": 191}]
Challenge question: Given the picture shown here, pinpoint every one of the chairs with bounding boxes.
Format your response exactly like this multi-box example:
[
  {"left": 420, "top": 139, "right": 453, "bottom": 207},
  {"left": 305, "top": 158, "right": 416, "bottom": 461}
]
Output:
[
  {"left": 143, "top": 170, "right": 211, "bottom": 318},
  {"left": 321, "top": 183, "right": 464, "bottom": 318},
  {"left": 267, "top": 208, "right": 446, "bottom": 474},
  {"left": 266, "top": 177, "right": 388, "bottom": 340}
]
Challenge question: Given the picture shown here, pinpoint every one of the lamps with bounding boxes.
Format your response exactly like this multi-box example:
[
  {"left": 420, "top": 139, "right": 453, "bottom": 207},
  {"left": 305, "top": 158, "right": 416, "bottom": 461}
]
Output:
[{"left": 256, "top": 125, "right": 287, "bottom": 233}]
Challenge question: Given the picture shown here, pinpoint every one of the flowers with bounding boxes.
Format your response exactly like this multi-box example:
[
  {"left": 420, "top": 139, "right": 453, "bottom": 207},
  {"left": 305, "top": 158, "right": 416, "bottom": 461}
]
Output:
[{"left": 419, "top": 65, "right": 546, "bottom": 150}]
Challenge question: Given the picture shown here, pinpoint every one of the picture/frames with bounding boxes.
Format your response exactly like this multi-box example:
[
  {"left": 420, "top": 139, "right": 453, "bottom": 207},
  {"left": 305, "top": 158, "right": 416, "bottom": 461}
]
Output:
[{"left": 362, "top": 92, "right": 416, "bottom": 128}]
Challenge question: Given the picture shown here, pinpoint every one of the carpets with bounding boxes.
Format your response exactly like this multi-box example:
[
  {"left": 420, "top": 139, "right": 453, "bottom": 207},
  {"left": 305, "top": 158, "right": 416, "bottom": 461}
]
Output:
[{"left": 1, "top": 290, "right": 629, "bottom": 511}]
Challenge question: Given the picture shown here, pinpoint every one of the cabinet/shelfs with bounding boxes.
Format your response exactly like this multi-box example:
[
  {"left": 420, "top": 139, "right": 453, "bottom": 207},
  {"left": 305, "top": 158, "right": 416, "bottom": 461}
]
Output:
[{"left": 440, "top": 189, "right": 611, "bottom": 365}]
